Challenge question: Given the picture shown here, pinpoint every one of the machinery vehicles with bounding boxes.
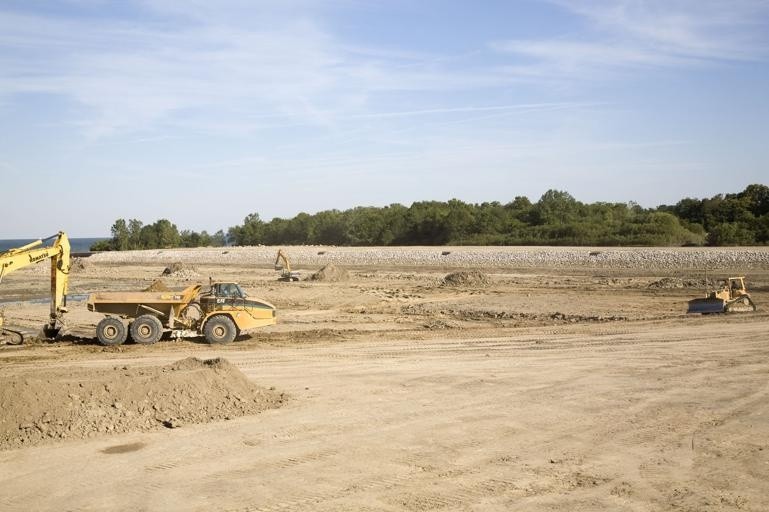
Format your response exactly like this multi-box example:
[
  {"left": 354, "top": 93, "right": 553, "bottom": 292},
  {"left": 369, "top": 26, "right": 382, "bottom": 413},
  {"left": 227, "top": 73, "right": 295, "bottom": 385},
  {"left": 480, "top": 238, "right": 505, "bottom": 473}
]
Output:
[
  {"left": 270, "top": 250, "right": 311, "bottom": 284},
  {"left": 1, "top": 229, "right": 278, "bottom": 346},
  {"left": 687, "top": 275, "right": 755, "bottom": 315}
]
